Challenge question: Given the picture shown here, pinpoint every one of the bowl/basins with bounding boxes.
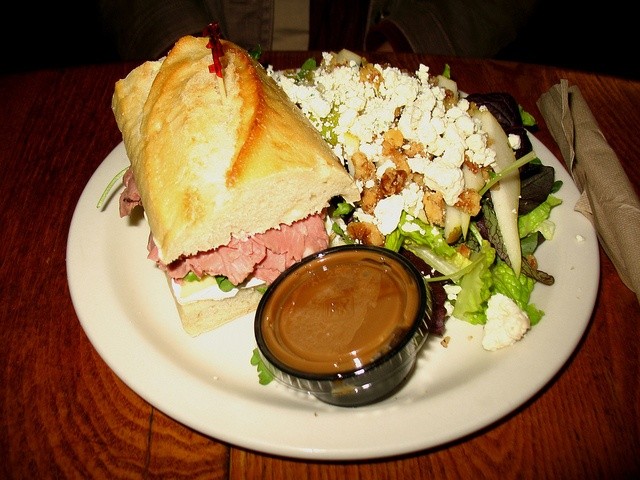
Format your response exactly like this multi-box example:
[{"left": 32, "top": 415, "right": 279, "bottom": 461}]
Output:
[{"left": 257, "top": 243, "right": 428, "bottom": 408}]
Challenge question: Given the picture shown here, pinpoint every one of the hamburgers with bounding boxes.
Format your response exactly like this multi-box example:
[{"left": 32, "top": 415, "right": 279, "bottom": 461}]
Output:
[{"left": 112, "top": 35, "right": 356, "bottom": 330}]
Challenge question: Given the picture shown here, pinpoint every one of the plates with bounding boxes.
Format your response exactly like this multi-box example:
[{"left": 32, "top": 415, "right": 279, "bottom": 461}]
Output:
[{"left": 64, "top": 70, "right": 603, "bottom": 461}]
